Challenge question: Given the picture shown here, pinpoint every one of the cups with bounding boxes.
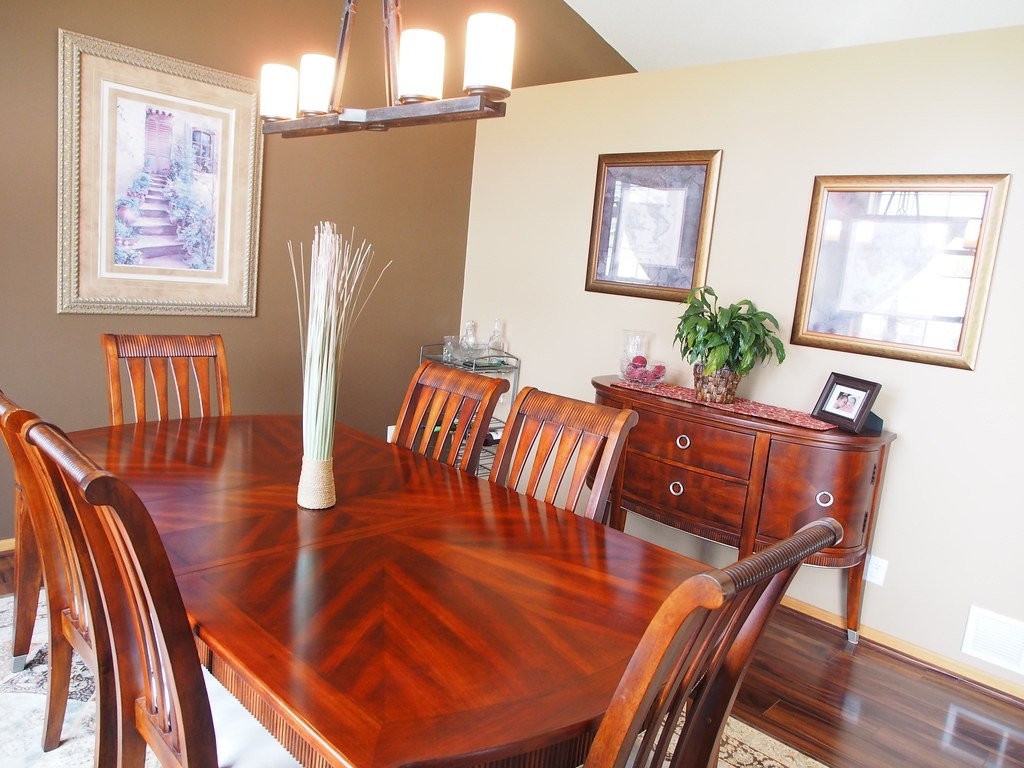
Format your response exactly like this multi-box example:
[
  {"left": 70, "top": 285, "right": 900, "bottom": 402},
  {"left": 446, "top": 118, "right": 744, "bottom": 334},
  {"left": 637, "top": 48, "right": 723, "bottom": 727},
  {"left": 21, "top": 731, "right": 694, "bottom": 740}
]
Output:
[{"left": 619, "top": 330, "right": 651, "bottom": 379}]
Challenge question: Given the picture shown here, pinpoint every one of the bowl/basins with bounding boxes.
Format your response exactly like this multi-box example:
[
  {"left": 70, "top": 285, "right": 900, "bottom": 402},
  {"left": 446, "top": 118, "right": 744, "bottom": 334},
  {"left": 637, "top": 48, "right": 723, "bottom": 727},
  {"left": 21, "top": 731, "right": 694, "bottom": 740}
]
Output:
[
  {"left": 620, "top": 358, "right": 669, "bottom": 387},
  {"left": 445, "top": 336, "right": 490, "bottom": 360}
]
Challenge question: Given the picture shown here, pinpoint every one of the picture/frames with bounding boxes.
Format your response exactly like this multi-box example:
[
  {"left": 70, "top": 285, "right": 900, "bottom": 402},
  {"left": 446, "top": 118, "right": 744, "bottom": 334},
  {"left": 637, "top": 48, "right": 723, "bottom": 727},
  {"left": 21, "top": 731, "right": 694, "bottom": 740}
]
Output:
[
  {"left": 57, "top": 28, "right": 265, "bottom": 317},
  {"left": 585, "top": 149, "right": 723, "bottom": 304},
  {"left": 811, "top": 372, "right": 882, "bottom": 436},
  {"left": 789, "top": 174, "right": 1012, "bottom": 372}
]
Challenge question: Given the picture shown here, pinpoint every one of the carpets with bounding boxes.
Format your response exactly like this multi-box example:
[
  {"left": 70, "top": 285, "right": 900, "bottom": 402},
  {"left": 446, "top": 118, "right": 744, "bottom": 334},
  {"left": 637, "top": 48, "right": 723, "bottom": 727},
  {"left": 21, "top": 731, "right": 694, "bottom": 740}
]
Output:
[{"left": 0, "top": 589, "right": 834, "bottom": 768}]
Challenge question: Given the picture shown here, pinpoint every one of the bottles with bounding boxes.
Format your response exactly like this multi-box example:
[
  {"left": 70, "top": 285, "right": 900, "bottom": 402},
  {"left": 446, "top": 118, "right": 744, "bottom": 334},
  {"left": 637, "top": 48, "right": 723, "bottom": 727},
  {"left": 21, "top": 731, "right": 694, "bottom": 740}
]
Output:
[
  {"left": 488, "top": 319, "right": 506, "bottom": 363},
  {"left": 461, "top": 321, "right": 476, "bottom": 347}
]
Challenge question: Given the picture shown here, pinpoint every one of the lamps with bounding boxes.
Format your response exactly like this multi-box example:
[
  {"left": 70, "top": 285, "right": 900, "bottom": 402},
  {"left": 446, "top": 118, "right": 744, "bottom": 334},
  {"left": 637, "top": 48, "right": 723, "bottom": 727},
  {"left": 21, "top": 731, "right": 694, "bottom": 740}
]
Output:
[{"left": 259, "top": 0, "right": 517, "bottom": 139}]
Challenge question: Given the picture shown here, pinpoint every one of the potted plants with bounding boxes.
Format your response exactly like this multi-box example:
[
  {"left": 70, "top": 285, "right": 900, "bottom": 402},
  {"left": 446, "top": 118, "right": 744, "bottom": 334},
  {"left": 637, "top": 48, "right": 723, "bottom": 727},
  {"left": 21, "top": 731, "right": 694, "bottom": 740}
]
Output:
[{"left": 673, "top": 285, "right": 785, "bottom": 404}]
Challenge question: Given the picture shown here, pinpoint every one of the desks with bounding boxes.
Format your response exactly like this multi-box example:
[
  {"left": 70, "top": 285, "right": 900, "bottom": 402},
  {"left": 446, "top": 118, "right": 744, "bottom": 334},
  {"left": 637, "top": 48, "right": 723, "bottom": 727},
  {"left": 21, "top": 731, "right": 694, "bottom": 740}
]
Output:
[
  {"left": 593, "top": 375, "right": 898, "bottom": 645},
  {"left": 59, "top": 413, "right": 744, "bottom": 768}
]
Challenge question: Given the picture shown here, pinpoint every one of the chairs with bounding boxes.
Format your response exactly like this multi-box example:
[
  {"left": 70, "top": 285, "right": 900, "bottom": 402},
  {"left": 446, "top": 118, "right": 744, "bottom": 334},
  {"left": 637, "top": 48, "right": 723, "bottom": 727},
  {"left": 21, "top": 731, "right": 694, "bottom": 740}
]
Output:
[
  {"left": 100, "top": 333, "right": 231, "bottom": 426},
  {"left": 583, "top": 516, "right": 845, "bottom": 768},
  {"left": 487, "top": 387, "right": 640, "bottom": 522},
  {"left": 391, "top": 360, "right": 510, "bottom": 475},
  {"left": 0, "top": 391, "right": 301, "bottom": 768}
]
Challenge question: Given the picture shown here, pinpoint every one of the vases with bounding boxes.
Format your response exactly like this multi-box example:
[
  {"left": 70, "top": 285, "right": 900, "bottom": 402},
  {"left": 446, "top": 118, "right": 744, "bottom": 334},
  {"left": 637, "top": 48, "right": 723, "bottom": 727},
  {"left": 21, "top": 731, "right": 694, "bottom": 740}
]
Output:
[{"left": 296, "top": 455, "right": 337, "bottom": 509}]
